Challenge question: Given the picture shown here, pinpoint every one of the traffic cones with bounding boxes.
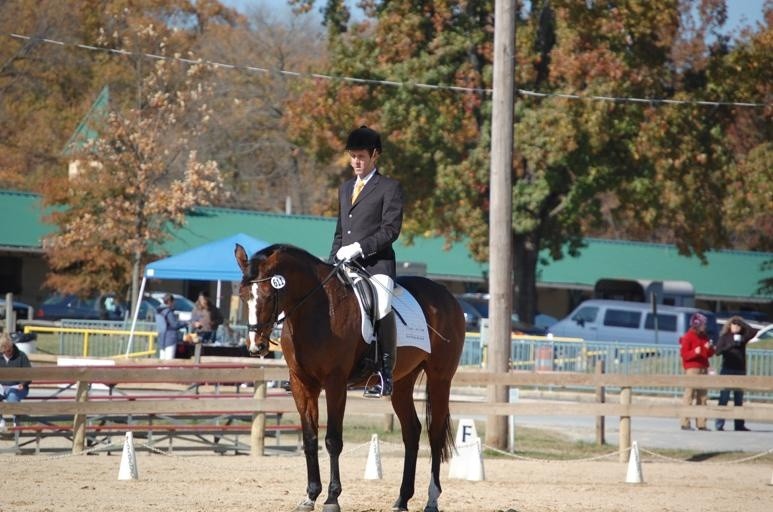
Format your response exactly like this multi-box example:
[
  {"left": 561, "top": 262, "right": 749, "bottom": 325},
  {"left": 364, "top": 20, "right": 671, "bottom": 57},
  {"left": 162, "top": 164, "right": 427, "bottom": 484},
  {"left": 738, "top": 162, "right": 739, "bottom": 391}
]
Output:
[
  {"left": 446, "top": 417, "right": 486, "bottom": 482},
  {"left": 361, "top": 434, "right": 384, "bottom": 481},
  {"left": 624, "top": 440, "right": 643, "bottom": 483},
  {"left": 116, "top": 431, "right": 139, "bottom": 481}
]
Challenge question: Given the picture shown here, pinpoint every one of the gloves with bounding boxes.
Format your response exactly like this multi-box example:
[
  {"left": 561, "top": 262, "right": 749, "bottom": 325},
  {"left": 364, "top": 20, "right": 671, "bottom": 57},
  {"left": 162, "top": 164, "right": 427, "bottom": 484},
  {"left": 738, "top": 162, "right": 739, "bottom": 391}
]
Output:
[{"left": 336, "top": 241, "right": 362, "bottom": 261}]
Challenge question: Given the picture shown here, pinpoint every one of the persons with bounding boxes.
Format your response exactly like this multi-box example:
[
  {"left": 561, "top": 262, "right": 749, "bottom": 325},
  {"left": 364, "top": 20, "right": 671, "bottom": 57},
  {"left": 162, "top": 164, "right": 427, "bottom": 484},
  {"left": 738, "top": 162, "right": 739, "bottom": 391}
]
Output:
[
  {"left": 191, "top": 291, "right": 223, "bottom": 343},
  {"left": 327, "top": 126, "right": 402, "bottom": 396},
  {"left": 1, "top": 333, "right": 31, "bottom": 427},
  {"left": 155, "top": 295, "right": 188, "bottom": 360},
  {"left": 716, "top": 316, "right": 755, "bottom": 431},
  {"left": 681, "top": 313, "right": 715, "bottom": 429}
]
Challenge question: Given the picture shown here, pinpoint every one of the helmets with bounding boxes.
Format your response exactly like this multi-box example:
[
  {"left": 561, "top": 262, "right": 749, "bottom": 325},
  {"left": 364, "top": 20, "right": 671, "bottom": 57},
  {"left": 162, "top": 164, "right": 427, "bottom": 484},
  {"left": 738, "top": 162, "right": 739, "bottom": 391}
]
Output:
[{"left": 345, "top": 126, "right": 381, "bottom": 149}]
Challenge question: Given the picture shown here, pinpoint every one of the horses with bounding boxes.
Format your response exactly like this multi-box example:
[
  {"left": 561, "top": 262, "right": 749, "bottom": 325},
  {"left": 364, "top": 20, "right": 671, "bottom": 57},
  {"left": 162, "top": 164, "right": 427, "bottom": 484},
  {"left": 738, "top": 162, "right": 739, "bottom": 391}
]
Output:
[{"left": 234, "top": 243, "right": 465, "bottom": 512}]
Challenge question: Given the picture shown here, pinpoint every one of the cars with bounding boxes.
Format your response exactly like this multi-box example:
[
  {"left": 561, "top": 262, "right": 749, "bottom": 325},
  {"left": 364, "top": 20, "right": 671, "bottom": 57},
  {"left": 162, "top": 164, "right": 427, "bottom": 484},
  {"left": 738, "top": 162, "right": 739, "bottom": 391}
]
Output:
[
  {"left": 713, "top": 310, "right": 773, "bottom": 345},
  {"left": 0, "top": 300, "right": 36, "bottom": 321},
  {"left": 143, "top": 292, "right": 194, "bottom": 323},
  {"left": 453, "top": 279, "right": 558, "bottom": 353},
  {"left": 36, "top": 292, "right": 160, "bottom": 336}
]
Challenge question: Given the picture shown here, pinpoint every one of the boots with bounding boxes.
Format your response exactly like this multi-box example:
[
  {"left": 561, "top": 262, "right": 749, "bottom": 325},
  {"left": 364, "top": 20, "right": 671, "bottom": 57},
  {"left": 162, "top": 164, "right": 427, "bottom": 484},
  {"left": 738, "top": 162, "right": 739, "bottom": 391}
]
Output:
[{"left": 362, "top": 310, "right": 396, "bottom": 392}]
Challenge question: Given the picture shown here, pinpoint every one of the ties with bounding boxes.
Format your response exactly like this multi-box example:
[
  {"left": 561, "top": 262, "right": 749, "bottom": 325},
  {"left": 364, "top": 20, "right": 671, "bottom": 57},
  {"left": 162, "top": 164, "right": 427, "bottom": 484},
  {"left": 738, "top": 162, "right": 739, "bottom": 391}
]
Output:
[{"left": 351, "top": 181, "right": 363, "bottom": 204}]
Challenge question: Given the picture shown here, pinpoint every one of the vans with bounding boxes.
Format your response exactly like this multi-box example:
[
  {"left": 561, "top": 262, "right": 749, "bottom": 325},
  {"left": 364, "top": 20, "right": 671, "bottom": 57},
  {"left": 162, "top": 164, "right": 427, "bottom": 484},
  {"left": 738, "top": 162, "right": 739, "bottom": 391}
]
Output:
[{"left": 547, "top": 299, "right": 719, "bottom": 362}]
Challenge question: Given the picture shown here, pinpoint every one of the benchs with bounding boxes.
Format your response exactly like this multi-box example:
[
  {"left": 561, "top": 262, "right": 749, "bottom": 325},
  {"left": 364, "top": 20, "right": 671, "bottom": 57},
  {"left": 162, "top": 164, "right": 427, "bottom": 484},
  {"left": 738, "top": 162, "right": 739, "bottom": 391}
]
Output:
[{"left": 0, "top": 362, "right": 326, "bottom": 456}]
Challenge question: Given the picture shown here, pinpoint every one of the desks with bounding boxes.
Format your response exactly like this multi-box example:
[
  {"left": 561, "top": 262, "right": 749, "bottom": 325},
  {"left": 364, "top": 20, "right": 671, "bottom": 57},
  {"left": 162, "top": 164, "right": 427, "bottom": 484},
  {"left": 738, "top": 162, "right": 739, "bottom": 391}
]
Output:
[{"left": 172, "top": 342, "right": 273, "bottom": 360}]
